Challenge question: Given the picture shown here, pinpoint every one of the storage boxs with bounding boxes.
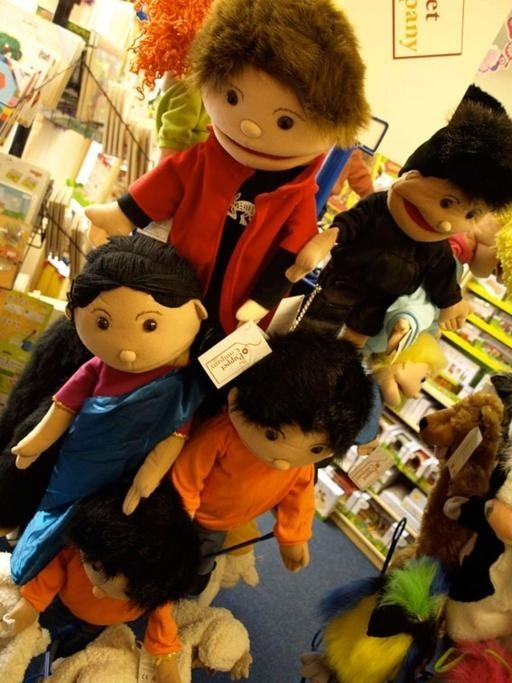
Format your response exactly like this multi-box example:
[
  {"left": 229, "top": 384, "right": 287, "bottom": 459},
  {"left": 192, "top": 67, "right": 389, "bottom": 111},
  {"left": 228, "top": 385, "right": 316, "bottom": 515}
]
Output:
[{"left": 0, "top": 287, "right": 65, "bottom": 417}]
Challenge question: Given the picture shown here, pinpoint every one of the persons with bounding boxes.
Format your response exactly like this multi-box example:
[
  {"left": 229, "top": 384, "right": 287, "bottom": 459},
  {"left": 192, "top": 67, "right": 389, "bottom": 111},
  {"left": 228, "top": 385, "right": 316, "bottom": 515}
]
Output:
[{"left": 1, "top": 0, "right": 511, "bottom": 682}]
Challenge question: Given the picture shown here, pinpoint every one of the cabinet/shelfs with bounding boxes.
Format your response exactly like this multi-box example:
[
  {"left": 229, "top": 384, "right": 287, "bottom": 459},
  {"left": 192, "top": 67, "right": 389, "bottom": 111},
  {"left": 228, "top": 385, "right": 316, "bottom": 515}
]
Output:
[{"left": 273, "top": 150, "right": 512, "bottom": 582}]
